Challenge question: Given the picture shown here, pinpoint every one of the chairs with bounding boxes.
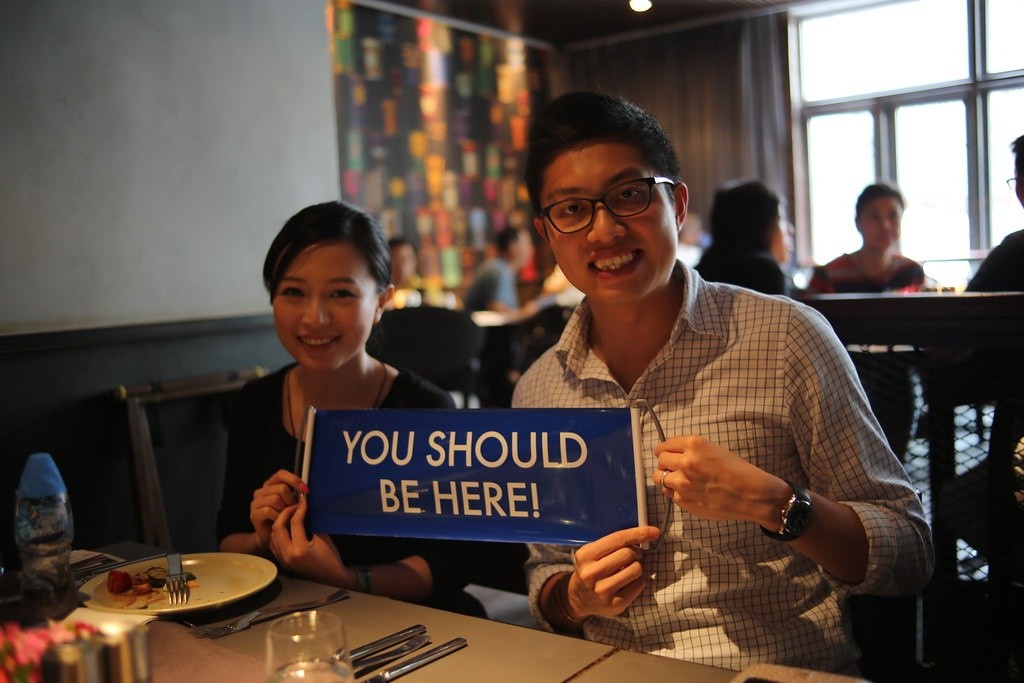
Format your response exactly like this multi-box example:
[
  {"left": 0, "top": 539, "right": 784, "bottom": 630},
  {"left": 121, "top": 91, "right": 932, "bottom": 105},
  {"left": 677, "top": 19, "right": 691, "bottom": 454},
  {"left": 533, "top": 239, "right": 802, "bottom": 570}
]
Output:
[{"left": 382, "top": 305, "right": 487, "bottom": 408}]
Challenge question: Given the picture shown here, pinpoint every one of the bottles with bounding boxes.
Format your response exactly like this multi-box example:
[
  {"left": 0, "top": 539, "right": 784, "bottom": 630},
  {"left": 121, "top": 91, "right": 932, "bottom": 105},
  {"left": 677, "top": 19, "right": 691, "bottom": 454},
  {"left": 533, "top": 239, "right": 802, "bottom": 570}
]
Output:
[{"left": 12, "top": 453, "right": 72, "bottom": 622}]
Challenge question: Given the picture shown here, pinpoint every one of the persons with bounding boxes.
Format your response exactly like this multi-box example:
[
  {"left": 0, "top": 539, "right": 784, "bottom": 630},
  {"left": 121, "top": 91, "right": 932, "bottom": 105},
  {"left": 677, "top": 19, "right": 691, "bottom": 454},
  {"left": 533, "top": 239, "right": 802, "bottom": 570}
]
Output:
[
  {"left": 510, "top": 92, "right": 935, "bottom": 678},
  {"left": 955, "top": 137, "right": 1023, "bottom": 577},
  {"left": 461, "top": 225, "right": 576, "bottom": 409},
  {"left": 384, "top": 237, "right": 442, "bottom": 296},
  {"left": 807, "top": 184, "right": 924, "bottom": 467},
  {"left": 694, "top": 178, "right": 794, "bottom": 295},
  {"left": 215, "top": 203, "right": 458, "bottom": 611}
]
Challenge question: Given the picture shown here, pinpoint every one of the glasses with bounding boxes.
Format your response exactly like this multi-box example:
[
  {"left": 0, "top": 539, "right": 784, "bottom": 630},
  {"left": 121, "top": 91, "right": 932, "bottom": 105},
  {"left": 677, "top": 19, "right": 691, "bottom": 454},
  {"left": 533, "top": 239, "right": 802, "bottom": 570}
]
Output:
[
  {"left": 1006, "top": 176, "right": 1022, "bottom": 188},
  {"left": 536, "top": 176, "right": 675, "bottom": 234}
]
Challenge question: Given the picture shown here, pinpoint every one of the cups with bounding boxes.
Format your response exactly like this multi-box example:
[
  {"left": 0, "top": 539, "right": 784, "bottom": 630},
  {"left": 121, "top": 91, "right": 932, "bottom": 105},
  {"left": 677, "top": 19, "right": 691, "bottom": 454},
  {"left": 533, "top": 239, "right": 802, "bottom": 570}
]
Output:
[{"left": 263, "top": 609, "right": 355, "bottom": 683}]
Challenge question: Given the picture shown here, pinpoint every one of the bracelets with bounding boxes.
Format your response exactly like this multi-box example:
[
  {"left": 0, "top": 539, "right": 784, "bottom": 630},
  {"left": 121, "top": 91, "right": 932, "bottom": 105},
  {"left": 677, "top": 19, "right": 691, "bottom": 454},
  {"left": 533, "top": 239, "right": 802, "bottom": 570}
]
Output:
[{"left": 555, "top": 582, "right": 583, "bottom": 624}]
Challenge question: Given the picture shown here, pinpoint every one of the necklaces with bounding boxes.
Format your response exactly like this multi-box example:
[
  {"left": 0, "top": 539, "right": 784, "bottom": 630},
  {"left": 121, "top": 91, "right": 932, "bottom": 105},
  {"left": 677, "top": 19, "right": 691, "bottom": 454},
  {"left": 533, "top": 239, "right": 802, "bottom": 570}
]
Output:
[{"left": 286, "top": 361, "right": 387, "bottom": 444}]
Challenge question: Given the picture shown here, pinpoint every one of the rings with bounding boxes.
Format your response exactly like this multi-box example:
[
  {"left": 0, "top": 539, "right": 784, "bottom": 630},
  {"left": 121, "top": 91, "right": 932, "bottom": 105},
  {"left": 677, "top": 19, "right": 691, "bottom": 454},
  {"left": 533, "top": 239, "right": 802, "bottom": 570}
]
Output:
[{"left": 659, "top": 471, "right": 670, "bottom": 487}]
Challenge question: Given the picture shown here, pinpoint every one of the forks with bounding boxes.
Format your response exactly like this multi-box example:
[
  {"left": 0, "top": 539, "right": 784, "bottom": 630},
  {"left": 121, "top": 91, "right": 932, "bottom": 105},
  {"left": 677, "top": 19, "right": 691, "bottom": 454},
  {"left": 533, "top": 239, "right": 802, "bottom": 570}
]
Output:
[
  {"left": 189, "top": 588, "right": 350, "bottom": 639},
  {"left": 167, "top": 553, "right": 187, "bottom": 607}
]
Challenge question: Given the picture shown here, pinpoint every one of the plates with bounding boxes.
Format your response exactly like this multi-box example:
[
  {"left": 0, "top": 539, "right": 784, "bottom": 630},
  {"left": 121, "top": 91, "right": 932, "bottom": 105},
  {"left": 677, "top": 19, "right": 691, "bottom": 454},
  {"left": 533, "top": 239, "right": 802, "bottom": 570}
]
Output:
[{"left": 78, "top": 553, "right": 278, "bottom": 616}]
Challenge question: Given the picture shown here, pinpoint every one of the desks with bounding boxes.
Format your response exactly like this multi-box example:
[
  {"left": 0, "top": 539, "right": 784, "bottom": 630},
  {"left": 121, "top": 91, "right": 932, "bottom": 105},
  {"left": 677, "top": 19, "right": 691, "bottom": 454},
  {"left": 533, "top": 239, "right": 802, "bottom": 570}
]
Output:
[
  {"left": 797, "top": 291, "right": 1024, "bottom": 577},
  {"left": 0, "top": 539, "right": 742, "bottom": 682}
]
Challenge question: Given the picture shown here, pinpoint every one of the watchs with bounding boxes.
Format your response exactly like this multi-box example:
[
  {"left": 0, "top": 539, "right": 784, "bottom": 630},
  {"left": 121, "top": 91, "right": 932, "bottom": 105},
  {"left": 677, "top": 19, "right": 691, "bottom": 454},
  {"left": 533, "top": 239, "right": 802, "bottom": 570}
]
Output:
[
  {"left": 351, "top": 564, "right": 371, "bottom": 594},
  {"left": 760, "top": 480, "right": 813, "bottom": 542}
]
